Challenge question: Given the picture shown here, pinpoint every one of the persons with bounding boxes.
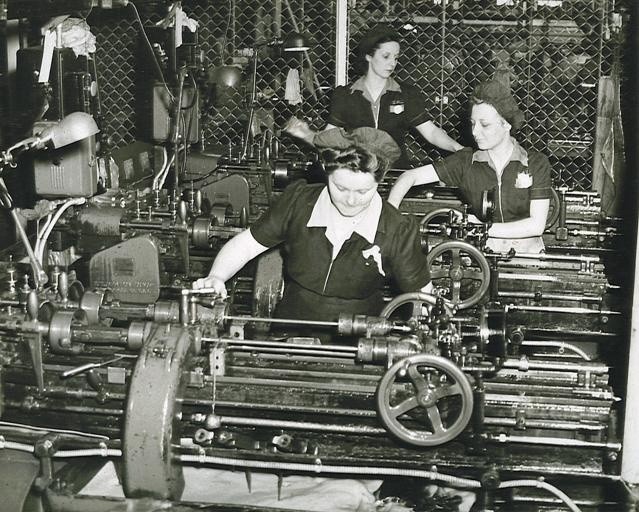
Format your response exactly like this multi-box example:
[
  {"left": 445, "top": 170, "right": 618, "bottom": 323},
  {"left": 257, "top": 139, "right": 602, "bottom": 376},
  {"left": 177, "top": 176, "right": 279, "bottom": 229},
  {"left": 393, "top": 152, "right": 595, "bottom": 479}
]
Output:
[
  {"left": 383, "top": 80, "right": 554, "bottom": 252},
  {"left": 285, "top": 25, "right": 462, "bottom": 179},
  {"left": 190, "top": 126, "right": 435, "bottom": 343}
]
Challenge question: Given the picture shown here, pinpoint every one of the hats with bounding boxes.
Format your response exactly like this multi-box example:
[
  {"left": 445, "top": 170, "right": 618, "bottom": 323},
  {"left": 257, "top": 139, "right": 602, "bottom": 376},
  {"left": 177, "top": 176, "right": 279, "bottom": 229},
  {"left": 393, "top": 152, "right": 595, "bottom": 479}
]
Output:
[
  {"left": 312, "top": 126, "right": 402, "bottom": 164},
  {"left": 468, "top": 80, "right": 526, "bottom": 137},
  {"left": 357, "top": 31, "right": 392, "bottom": 55}
]
